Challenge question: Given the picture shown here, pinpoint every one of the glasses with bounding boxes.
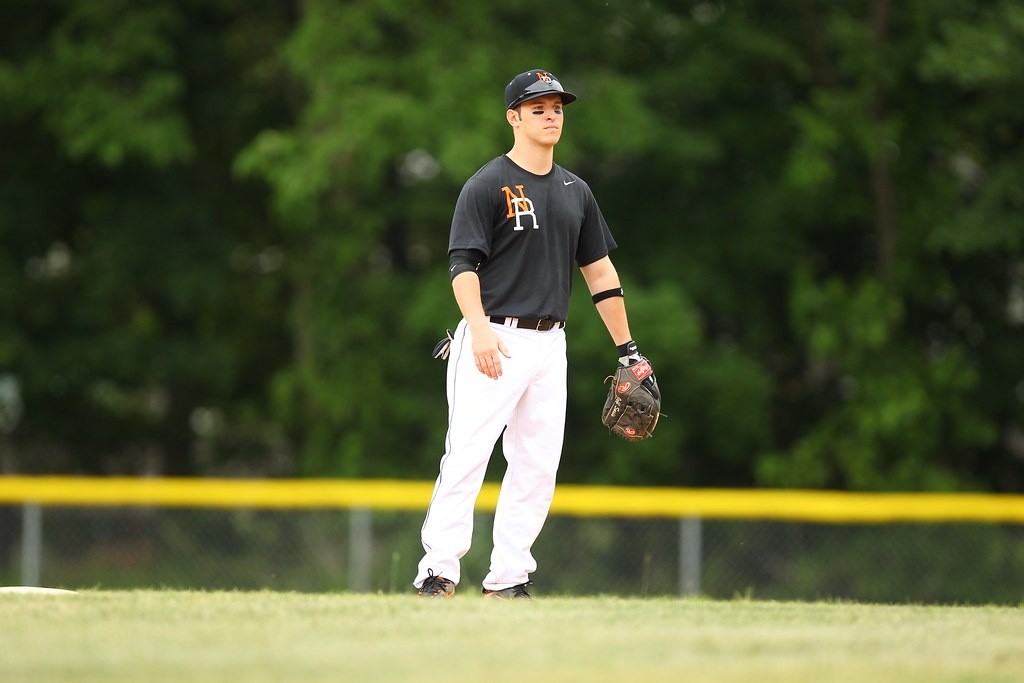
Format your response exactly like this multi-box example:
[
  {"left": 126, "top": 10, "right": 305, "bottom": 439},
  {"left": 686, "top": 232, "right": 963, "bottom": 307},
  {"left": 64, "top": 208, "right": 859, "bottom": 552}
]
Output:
[{"left": 506, "top": 80, "right": 564, "bottom": 110}]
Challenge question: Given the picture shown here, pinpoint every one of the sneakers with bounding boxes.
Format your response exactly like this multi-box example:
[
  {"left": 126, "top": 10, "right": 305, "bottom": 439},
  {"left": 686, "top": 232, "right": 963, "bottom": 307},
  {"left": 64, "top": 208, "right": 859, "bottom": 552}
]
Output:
[
  {"left": 418, "top": 568, "right": 455, "bottom": 599},
  {"left": 482, "top": 579, "right": 533, "bottom": 600}
]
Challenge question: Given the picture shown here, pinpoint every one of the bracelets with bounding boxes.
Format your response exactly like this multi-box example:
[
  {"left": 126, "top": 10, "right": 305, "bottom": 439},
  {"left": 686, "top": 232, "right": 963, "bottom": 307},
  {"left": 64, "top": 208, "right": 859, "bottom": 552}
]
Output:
[{"left": 591, "top": 287, "right": 624, "bottom": 305}]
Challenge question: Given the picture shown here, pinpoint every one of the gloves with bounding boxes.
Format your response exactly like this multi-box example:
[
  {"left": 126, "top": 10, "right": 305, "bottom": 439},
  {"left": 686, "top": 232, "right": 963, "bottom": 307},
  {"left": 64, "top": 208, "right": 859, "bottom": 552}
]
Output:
[{"left": 617, "top": 340, "right": 659, "bottom": 400}]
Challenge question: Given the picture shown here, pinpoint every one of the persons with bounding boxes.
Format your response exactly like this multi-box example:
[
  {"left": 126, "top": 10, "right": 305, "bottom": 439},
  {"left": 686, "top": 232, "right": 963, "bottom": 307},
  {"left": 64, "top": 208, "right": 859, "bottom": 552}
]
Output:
[{"left": 411, "top": 68, "right": 661, "bottom": 602}]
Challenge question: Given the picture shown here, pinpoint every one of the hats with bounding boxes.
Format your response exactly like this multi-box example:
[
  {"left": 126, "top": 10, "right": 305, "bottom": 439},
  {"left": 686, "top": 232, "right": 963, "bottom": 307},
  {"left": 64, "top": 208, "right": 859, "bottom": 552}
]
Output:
[{"left": 505, "top": 69, "right": 577, "bottom": 112}]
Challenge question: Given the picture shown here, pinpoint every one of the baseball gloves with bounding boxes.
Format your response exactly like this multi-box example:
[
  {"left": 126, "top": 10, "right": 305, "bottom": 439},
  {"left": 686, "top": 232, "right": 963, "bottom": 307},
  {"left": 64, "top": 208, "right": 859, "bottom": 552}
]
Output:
[{"left": 602, "top": 357, "right": 662, "bottom": 442}]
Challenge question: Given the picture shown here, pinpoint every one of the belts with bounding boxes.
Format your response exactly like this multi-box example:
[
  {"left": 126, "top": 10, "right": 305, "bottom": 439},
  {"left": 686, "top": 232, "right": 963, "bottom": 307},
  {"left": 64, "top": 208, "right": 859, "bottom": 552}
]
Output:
[{"left": 490, "top": 315, "right": 564, "bottom": 332}]
